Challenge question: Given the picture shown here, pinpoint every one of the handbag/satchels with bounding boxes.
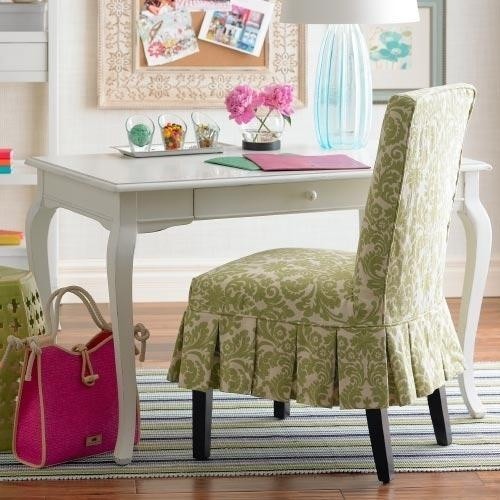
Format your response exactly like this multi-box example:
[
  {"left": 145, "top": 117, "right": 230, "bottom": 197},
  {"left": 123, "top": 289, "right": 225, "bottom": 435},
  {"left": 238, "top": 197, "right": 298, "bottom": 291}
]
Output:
[{"left": 13, "top": 285, "right": 141, "bottom": 469}]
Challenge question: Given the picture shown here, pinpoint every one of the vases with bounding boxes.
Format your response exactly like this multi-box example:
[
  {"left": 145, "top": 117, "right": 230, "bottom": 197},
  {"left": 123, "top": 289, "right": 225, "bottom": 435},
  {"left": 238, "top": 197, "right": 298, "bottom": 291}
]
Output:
[{"left": 241, "top": 106, "right": 285, "bottom": 150}]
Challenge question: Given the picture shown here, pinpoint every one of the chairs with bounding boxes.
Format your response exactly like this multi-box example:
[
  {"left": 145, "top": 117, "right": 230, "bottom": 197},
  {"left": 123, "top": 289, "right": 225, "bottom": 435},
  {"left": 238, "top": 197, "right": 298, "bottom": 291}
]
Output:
[{"left": 167, "top": 82, "right": 476, "bottom": 482}]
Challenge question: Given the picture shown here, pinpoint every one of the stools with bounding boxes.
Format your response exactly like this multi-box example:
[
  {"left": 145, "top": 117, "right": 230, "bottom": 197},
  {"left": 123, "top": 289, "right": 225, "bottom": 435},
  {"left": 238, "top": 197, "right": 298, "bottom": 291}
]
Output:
[{"left": 0, "top": 265, "right": 48, "bottom": 453}]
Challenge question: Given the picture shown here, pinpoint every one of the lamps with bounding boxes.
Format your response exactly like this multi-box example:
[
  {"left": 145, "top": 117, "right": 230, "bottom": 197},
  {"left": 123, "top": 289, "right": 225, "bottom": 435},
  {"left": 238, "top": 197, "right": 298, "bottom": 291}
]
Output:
[{"left": 280, "top": 0, "right": 421, "bottom": 150}]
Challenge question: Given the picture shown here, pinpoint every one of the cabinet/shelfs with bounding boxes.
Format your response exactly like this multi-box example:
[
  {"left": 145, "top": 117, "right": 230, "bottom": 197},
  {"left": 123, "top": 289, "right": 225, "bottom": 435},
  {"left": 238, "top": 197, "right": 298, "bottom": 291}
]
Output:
[
  {"left": 0, "top": 1, "right": 56, "bottom": 272},
  {"left": 23, "top": 141, "right": 493, "bottom": 464}
]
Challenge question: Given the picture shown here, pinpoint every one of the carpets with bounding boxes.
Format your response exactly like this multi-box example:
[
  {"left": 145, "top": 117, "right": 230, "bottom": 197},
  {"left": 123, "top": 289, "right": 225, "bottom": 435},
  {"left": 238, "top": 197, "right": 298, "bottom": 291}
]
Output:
[{"left": 1, "top": 361, "right": 500, "bottom": 482}]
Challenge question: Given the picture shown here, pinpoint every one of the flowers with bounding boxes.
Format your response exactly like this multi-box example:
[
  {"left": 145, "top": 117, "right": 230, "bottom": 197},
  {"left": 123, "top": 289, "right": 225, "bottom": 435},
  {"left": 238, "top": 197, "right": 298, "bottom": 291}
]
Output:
[{"left": 223, "top": 83, "right": 295, "bottom": 142}]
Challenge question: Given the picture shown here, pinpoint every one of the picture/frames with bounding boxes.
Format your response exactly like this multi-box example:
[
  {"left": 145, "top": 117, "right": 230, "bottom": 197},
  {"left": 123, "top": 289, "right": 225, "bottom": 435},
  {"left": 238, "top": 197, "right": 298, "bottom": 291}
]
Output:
[
  {"left": 96, "top": 0, "right": 306, "bottom": 110},
  {"left": 366, "top": 2, "right": 447, "bottom": 104}
]
